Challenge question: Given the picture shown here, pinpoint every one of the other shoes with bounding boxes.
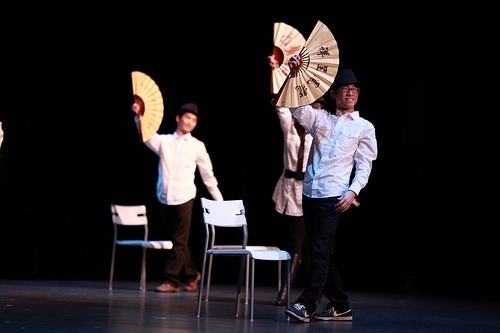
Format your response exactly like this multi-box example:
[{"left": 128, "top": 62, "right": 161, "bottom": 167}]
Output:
[
  {"left": 155, "top": 282, "right": 180, "bottom": 293},
  {"left": 184, "top": 272, "right": 200, "bottom": 291}
]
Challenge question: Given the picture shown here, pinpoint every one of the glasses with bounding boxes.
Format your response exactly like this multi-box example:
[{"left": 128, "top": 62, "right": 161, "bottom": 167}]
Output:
[{"left": 338, "top": 88, "right": 360, "bottom": 93}]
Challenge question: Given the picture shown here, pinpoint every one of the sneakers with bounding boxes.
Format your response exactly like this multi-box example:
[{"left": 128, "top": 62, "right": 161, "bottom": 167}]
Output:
[
  {"left": 285, "top": 303, "right": 311, "bottom": 323},
  {"left": 313, "top": 305, "right": 353, "bottom": 321}
]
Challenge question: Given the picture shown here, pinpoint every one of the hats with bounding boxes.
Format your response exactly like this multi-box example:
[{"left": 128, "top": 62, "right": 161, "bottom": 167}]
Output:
[
  {"left": 179, "top": 103, "right": 204, "bottom": 122},
  {"left": 332, "top": 68, "right": 358, "bottom": 88}
]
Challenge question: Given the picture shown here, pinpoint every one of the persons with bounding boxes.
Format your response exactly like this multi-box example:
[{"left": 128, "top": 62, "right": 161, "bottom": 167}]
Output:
[
  {"left": 284, "top": 55, "right": 379, "bottom": 322},
  {"left": 132, "top": 102, "right": 224, "bottom": 292},
  {"left": 268, "top": 56, "right": 327, "bottom": 306}
]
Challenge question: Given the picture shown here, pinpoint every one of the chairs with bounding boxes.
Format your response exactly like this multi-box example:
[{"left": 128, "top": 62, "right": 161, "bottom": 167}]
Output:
[
  {"left": 108, "top": 204, "right": 173, "bottom": 293},
  {"left": 195, "top": 198, "right": 293, "bottom": 321}
]
